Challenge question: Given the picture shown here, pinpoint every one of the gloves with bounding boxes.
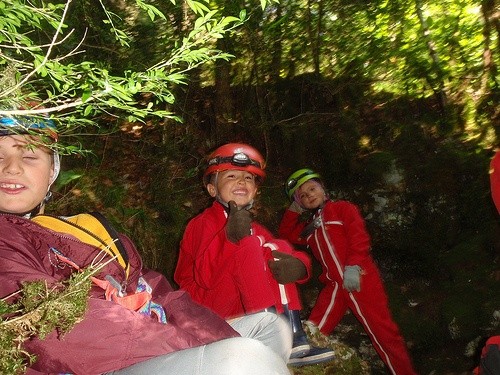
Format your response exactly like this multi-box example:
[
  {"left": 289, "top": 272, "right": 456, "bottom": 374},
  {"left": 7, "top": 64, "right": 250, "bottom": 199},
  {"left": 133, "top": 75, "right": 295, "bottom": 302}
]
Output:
[
  {"left": 267, "top": 250, "right": 307, "bottom": 283},
  {"left": 289, "top": 201, "right": 307, "bottom": 214},
  {"left": 226, "top": 200, "right": 253, "bottom": 243},
  {"left": 343, "top": 265, "right": 362, "bottom": 293}
]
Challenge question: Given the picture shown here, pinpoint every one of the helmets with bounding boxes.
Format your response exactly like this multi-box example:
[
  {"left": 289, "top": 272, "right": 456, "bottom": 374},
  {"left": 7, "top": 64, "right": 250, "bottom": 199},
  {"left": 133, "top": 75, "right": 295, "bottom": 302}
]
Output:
[
  {"left": 0, "top": 94, "right": 60, "bottom": 201},
  {"left": 284, "top": 169, "right": 321, "bottom": 202},
  {"left": 203, "top": 143, "right": 267, "bottom": 191}
]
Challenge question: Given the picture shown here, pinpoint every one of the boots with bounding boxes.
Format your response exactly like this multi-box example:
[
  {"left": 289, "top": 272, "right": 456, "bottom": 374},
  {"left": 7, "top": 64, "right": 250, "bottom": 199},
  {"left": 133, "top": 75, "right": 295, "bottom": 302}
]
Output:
[
  {"left": 259, "top": 303, "right": 308, "bottom": 360},
  {"left": 281, "top": 304, "right": 335, "bottom": 367}
]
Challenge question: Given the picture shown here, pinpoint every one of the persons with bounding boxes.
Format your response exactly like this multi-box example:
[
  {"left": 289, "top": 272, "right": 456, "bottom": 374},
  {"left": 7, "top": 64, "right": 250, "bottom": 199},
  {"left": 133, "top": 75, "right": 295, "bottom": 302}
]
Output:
[
  {"left": 277, "top": 167, "right": 420, "bottom": 375},
  {"left": 172, "top": 142, "right": 335, "bottom": 366},
  {"left": 0, "top": 91, "right": 292, "bottom": 375}
]
